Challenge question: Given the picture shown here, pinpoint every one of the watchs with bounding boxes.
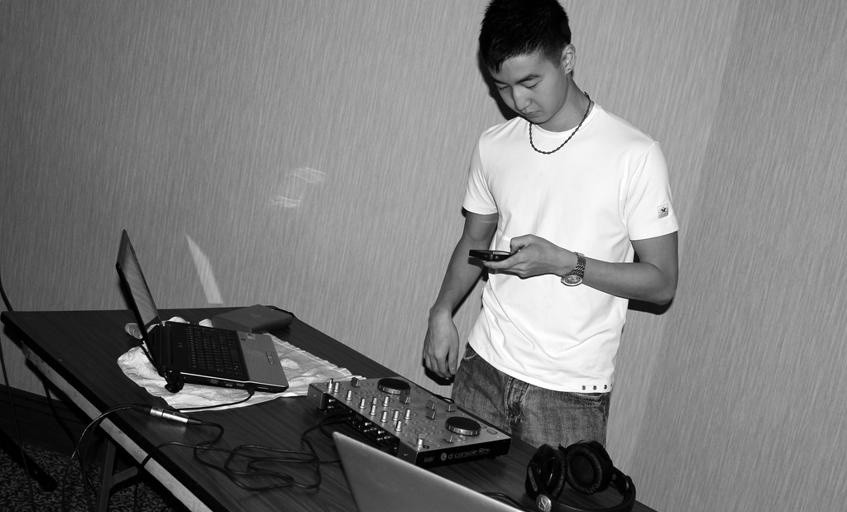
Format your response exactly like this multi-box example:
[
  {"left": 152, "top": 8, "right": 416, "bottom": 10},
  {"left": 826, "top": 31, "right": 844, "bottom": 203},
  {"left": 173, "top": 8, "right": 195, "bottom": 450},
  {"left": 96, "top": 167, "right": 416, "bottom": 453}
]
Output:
[{"left": 560, "top": 252, "right": 587, "bottom": 286}]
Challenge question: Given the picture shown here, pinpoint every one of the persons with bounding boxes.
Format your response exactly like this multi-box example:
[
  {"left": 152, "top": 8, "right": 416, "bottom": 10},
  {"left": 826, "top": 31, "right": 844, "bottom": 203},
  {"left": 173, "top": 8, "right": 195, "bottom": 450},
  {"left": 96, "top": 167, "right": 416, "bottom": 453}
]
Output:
[{"left": 422, "top": 0, "right": 678, "bottom": 459}]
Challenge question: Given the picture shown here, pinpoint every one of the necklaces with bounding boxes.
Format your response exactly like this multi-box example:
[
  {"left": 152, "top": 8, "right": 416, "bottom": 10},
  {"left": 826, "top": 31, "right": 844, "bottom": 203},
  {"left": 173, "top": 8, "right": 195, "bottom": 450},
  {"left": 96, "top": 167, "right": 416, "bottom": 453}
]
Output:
[{"left": 528, "top": 93, "right": 592, "bottom": 154}]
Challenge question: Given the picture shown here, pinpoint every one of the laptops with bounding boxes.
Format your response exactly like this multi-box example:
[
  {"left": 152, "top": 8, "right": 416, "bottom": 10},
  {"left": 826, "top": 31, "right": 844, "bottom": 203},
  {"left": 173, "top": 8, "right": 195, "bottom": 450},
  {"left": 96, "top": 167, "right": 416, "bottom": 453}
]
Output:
[
  {"left": 330, "top": 432, "right": 524, "bottom": 511},
  {"left": 116, "top": 230, "right": 289, "bottom": 393}
]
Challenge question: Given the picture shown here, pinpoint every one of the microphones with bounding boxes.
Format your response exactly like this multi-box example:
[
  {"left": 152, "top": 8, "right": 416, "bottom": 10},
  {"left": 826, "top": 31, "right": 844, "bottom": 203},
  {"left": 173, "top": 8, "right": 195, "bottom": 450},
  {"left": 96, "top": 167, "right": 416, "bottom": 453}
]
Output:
[{"left": 135, "top": 404, "right": 204, "bottom": 426}]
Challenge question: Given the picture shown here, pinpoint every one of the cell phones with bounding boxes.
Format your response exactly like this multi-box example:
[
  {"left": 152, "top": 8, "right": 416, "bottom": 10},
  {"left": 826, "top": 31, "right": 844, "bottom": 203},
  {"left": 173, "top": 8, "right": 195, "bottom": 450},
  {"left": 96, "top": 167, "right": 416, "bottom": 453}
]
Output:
[{"left": 469, "top": 248, "right": 515, "bottom": 261}]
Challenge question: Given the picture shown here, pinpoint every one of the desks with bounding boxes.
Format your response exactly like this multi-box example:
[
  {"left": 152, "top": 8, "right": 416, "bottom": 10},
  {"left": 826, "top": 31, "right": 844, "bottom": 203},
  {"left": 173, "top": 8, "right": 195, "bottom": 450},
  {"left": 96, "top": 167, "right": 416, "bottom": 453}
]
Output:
[{"left": 0, "top": 304, "right": 658, "bottom": 512}]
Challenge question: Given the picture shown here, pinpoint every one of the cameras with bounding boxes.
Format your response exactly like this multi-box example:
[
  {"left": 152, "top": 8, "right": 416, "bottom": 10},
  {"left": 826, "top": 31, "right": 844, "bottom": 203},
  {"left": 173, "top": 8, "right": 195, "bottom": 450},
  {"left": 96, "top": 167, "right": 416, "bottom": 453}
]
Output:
[{"left": 529, "top": 439, "right": 636, "bottom": 512}]
